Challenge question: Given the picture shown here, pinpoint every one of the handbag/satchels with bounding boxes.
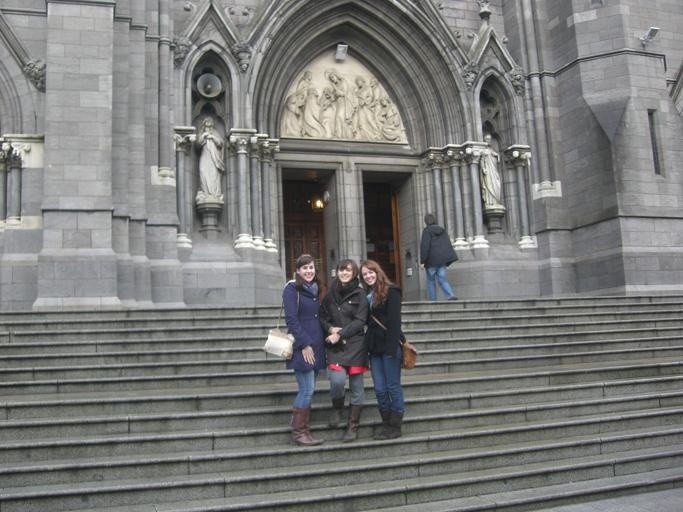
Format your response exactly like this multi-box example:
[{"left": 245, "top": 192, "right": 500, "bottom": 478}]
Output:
[
  {"left": 262, "top": 329, "right": 295, "bottom": 360},
  {"left": 402, "top": 342, "right": 417, "bottom": 370}
]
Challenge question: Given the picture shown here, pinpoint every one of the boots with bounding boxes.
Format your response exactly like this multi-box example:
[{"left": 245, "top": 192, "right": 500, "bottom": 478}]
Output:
[
  {"left": 329, "top": 395, "right": 345, "bottom": 426},
  {"left": 288, "top": 408, "right": 324, "bottom": 446},
  {"left": 342, "top": 403, "right": 363, "bottom": 442},
  {"left": 373, "top": 408, "right": 405, "bottom": 440}
]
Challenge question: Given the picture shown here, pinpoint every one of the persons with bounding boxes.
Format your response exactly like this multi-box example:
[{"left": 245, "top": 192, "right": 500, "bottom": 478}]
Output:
[
  {"left": 481, "top": 132, "right": 505, "bottom": 214},
  {"left": 419, "top": 214, "right": 460, "bottom": 303},
  {"left": 195, "top": 115, "right": 227, "bottom": 204},
  {"left": 358, "top": 259, "right": 412, "bottom": 444},
  {"left": 320, "top": 258, "right": 368, "bottom": 442},
  {"left": 282, "top": 253, "right": 325, "bottom": 445},
  {"left": 283, "top": 67, "right": 407, "bottom": 144}
]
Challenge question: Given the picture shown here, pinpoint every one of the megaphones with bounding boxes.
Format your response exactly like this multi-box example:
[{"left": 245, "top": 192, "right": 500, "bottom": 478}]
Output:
[{"left": 197, "top": 73, "right": 222, "bottom": 98}]
